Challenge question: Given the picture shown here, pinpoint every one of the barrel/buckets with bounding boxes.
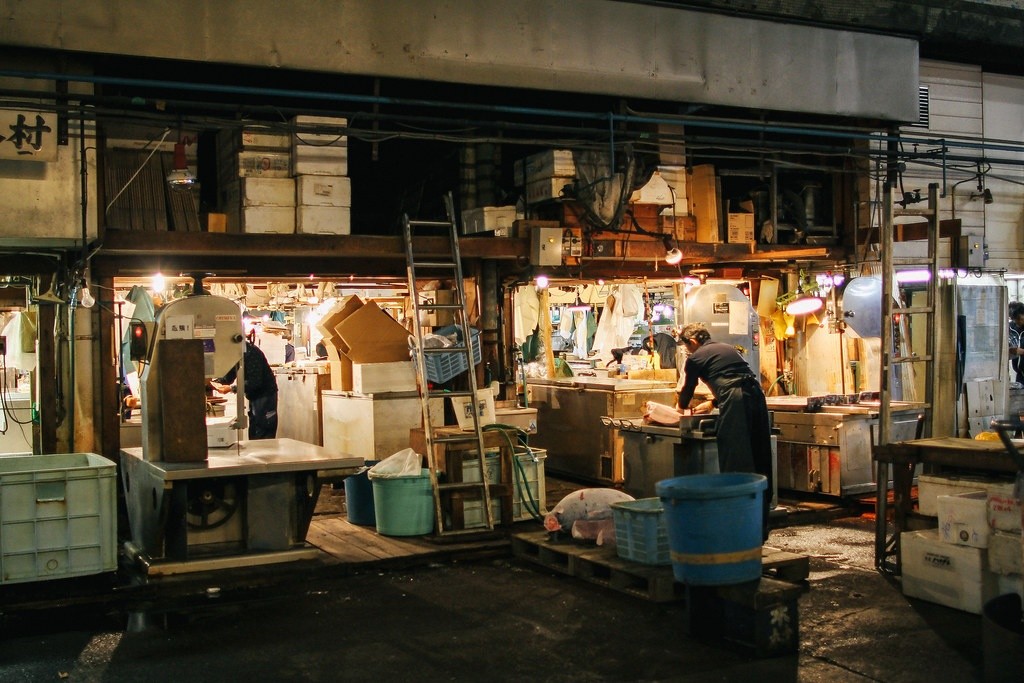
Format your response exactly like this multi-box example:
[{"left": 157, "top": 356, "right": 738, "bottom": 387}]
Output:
[
  {"left": 655, "top": 473, "right": 769, "bottom": 586},
  {"left": 982, "top": 591, "right": 1024, "bottom": 683},
  {"left": 343, "top": 461, "right": 434, "bottom": 536}
]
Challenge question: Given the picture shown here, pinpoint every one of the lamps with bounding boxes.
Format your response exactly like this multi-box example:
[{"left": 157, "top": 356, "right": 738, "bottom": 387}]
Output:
[
  {"left": 968, "top": 188, "right": 994, "bottom": 204},
  {"left": 787, "top": 296, "right": 822, "bottom": 314},
  {"left": 566, "top": 285, "right": 591, "bottom": 311}
]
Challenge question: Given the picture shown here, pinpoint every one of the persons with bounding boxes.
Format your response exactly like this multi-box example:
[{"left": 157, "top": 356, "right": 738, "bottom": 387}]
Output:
[
  {"left": 285, "top": 342, "right": 295, "bottom": 364},
  {"left": 676, "top": 322, "right": 773, "bottom": 547},
  {"left": 209, "top": 341, "right": 278, "bottom": 440},
  {"left": 1008, "top": 301, "right": 1024, "bottom": 438}
]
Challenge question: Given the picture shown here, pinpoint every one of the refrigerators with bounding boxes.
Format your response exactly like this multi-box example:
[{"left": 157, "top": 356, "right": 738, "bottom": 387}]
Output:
[{"left": 321, "top": 390, "right": 445, "bottom": 461}]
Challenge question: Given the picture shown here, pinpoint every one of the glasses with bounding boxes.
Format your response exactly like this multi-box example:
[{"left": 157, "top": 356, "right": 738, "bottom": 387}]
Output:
[{"left": 1009, "top": 316, "right": 1021, "bottom": 321}]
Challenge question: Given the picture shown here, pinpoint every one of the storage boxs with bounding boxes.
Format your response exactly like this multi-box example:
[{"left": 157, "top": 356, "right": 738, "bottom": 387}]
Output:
[
  {"left": 0, "top": 452, "right": 117, "bottom": 585},
  {"left": 609, "top": 495, "right": 673, "bottom": 567},
  {"left": 459, "top": 148, "right": 577, "bottom": 235},
  {"left": 238, "top": 113, "right": 351, "bottom": 234},
  {"left": 409, "top": 323, "right": 483, "bottom": 384},
  {"left": 965, "top": 379, "right": 1006, "bottom": 438},
  {"left": 722, "top": 199, "right": 755, "bottom": 253},
  {"left": 206, "top": 416, "right": 238, "bottom": 447},
  {"left": 898, "top": 473, "right": 1024, "bottom": 616},
  {"left": 751, "top": 274, "right": 782, "bottom": 320},
  {"left": 316, "top": 294, "right": 421, "bottom": 394},
  {"left": 444, "top": 444, "right": 548, "bottom": 530}
]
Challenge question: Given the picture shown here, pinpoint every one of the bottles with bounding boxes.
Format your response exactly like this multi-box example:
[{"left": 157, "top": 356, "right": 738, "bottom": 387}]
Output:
[{"left": 620, "top": 361, "right": 627, "bottom": 375}]
